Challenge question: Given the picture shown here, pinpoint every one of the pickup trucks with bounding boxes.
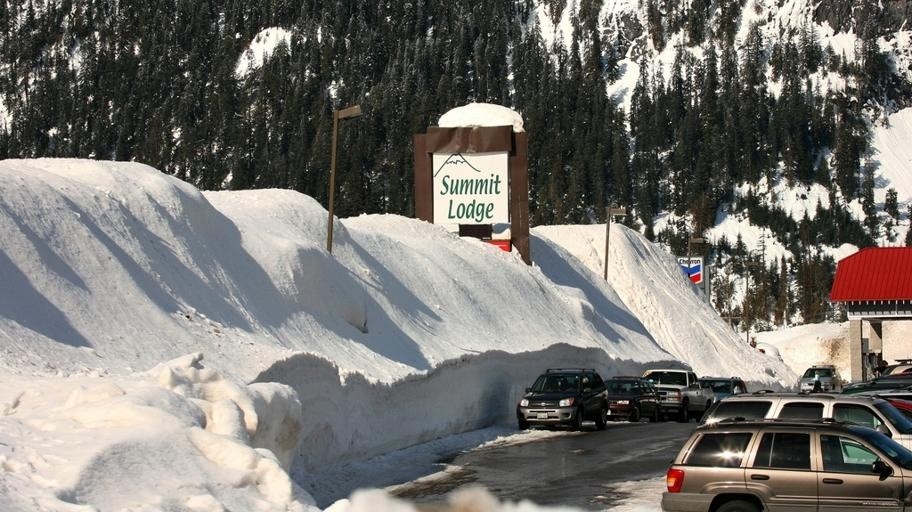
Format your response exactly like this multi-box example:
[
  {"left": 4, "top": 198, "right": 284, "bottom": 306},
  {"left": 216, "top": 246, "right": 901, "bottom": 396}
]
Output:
[{"left": 643, "top": 369, "right": 714, "bottom": 422}]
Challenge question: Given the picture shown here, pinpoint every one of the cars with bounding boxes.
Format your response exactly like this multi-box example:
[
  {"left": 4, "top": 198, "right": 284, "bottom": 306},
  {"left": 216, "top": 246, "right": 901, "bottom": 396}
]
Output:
[
  {"left": 842, "top": 364, "right": 912, "bottom": 392},
  {"left": 799, "top": 367, "right": 843, "bottom": 391},
  {"left": 603, "top": 376, "right": 660, "bottom": 422}
]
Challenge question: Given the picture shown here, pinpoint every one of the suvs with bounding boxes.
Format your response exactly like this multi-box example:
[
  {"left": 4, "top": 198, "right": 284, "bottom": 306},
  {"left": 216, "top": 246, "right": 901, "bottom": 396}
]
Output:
[
  {"left": 699, "top": 375, "right": 747, "bottom": 402},
  {"left": 516, "top": 368, "right": 609, "bottom": 430},
  {"left": 661, "top": 394, "right": 912, "bottom": 510}
]
